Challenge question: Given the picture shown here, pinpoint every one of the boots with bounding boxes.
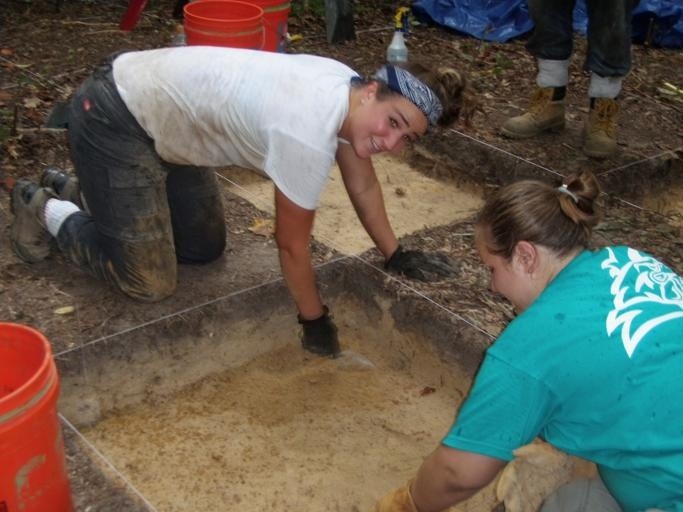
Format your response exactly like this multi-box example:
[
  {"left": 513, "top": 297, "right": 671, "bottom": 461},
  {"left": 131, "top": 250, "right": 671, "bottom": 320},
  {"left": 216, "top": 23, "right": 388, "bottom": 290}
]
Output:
[
  {"left": 500, "top": 89, "right": 565, "bottom": 140},
  {"left": 582, "top": 98, "right": 618, "bottom": 159}
]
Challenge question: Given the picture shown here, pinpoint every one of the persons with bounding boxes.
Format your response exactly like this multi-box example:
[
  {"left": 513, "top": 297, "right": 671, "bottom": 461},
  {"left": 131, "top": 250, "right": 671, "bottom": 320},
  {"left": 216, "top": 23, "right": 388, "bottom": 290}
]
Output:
[
  {"left": 5, "top": 45, "right": 466, "bottom": 357},
  {"left": 499, "top": 2, "right": 639, "bottom": 160},
  {"left": 365, "top": 170, "right": 681, "bottom": 511}
]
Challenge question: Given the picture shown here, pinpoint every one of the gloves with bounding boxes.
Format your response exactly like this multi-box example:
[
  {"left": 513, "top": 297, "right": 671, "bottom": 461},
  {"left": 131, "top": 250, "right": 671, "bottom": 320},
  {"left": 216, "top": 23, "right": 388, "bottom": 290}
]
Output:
[
  {"left": 296, "top": 306, "right": 340, "bottom": 360},
  {"left": 384, "top": 244, "right": 457, "bottom": 285},
  {"left": 375, "top": 476, "right": 454, "bottom": 512},
  {"left": 494, "top": 445, "right": 578, "bottom": 512}
]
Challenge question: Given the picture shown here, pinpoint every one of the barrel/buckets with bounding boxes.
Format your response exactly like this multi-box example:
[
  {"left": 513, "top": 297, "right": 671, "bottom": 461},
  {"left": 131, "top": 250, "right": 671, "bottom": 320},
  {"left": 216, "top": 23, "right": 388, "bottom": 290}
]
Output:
[
  {"left": 0, "top": 322, "right": 71, "bottom": 512},
  {"left": 182, "top": 0, "right": 266, "bottom": 47},
  {"left": 261, "top": 0, "right": 291, "bottom": 53}
]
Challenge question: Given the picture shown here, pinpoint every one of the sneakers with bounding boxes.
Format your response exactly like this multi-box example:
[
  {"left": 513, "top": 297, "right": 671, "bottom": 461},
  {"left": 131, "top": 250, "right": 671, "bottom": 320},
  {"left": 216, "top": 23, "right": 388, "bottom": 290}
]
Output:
[
  {"left": 40, "top": 165, "right": 80, "bottom": 204},
  {"left": 7, "top": 179, "right": 58, "bottom": 265}
]
